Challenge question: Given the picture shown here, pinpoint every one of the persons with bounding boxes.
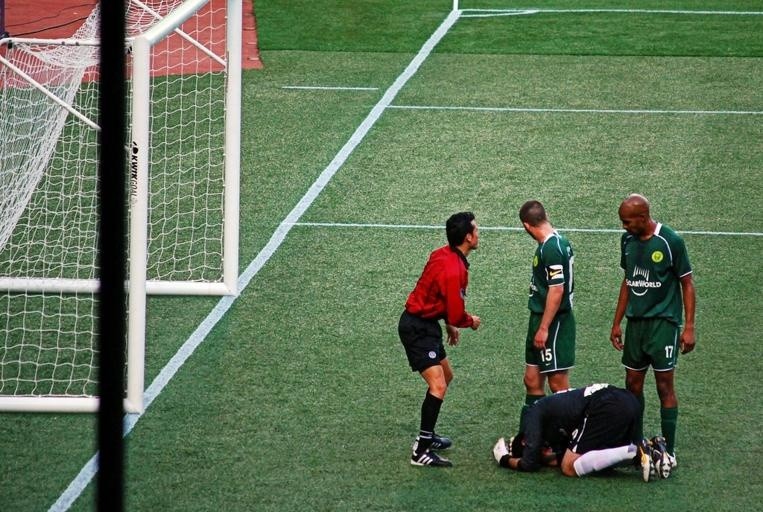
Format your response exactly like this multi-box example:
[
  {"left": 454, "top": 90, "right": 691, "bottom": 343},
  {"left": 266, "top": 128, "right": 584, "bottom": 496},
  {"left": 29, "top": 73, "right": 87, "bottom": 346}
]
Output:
[
  {"left": 518, "top": 199, "right": 577, "bottom": 409},
  {"left": 492, "top": 382, "right": 672, "bottom": 484},
  {"left": 397, "top": 211, "right": 482, "bottom": 469},
  {"left": 608, "top": 192, "right": 696, "bottom": 475}
]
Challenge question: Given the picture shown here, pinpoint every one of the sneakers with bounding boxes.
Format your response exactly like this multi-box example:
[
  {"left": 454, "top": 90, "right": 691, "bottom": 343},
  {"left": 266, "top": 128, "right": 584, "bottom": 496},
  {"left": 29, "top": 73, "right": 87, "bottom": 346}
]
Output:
[
  {"left": 633, "top": 437, "right": 650, "bottom": 483},
  {"left": 411, "top": 447, "right": 454, "bottom": 468},
  {"left": 669, "top": 451, "right": 678, "bottom": 466},
  {"left": 651, "top": 434, "right": 672, "bottom": 479},
  {"left": 411, "top": 437, "right": 454, "bottom": 451}
]
